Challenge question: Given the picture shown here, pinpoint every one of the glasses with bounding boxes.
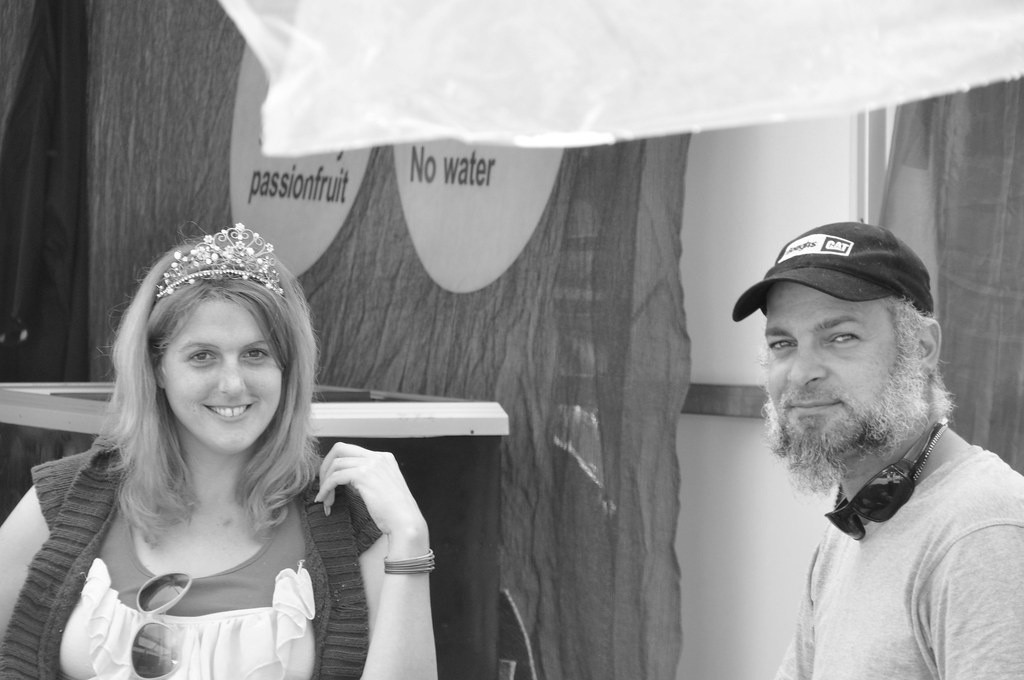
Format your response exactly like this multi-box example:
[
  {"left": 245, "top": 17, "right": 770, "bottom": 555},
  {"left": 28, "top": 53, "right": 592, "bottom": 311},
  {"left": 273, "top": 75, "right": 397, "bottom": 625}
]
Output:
[
  {"left": 132, "top": 573, "right": 193, "bottom": 680},
  {"left": 823, "top": 419, "right": 939, "bottom": 540}
]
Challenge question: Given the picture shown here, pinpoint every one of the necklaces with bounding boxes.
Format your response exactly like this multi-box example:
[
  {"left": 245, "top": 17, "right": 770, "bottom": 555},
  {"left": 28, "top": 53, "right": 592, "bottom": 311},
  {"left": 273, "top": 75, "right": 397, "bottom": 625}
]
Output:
[{"left": 904, "top": 425, "right": 950, "bottom": 482}]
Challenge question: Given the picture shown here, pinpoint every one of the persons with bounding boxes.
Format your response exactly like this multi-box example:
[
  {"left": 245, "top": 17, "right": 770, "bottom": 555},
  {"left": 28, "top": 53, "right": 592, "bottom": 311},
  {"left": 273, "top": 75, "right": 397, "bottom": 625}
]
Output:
[
  {"left": 0, "top": 221, "right": 442, "bottom": 680},
  {"left": 731, "top": 223, "right": 1022, "bottom": 680}
]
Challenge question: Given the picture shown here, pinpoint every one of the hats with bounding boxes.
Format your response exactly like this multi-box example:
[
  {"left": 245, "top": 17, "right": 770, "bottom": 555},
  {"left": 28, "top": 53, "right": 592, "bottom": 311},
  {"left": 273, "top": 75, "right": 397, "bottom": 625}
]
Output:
[{"left": 731, "top": 223, "right": 934, "bottom": 322}]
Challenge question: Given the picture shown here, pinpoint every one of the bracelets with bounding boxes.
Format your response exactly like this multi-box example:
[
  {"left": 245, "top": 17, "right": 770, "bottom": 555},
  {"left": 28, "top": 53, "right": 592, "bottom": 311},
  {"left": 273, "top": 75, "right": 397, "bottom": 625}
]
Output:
[{"left": 385, "top": 550, "right": 435, "bottom": 574}]
